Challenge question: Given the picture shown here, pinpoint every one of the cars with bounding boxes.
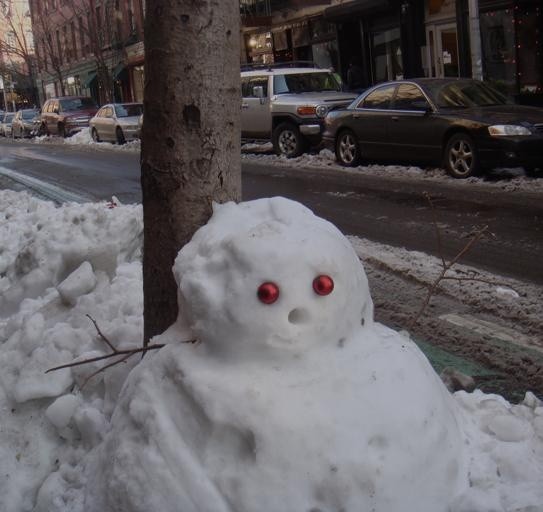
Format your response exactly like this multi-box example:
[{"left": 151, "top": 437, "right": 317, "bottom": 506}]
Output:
[
  {"left": 11, "top": 108, "right": 42, "bottom": 139},
  {"left": 0, "top": 110, "right": 16, "bottom": 138},
  {"left": 88, "top": 102, "right": 145, "bottom": 145},
  {"left": 319, "top": 77, "right": 543, "bottom": 179}
]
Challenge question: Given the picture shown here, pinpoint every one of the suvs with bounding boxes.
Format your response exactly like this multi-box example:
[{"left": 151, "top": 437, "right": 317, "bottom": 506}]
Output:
[
  {"left": 240, "top": 61, "right": 360, "bottom": 159},
  {"left": 41, "top": 96, "right": 99, "bottom": 138}
]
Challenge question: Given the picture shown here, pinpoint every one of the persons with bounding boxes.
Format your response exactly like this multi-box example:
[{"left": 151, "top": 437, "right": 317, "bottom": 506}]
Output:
[{"left": 325, "top": 66, "right": 342, "bottom": 89}]
